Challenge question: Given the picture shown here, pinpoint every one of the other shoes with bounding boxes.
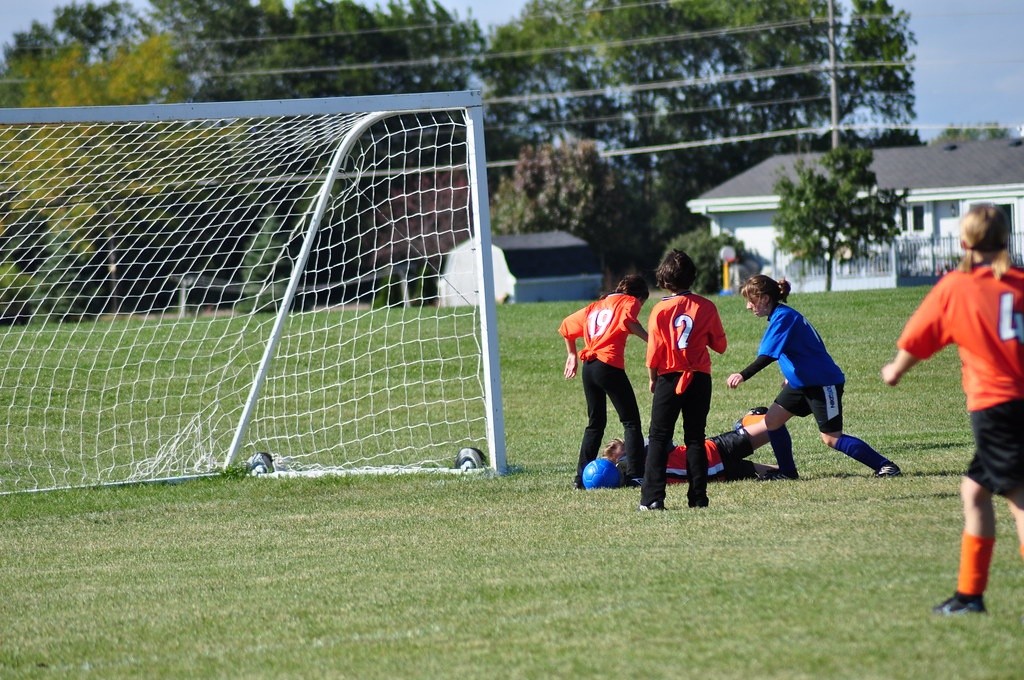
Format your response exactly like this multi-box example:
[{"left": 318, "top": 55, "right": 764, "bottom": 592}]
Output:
[
  {"left": 639, "top": 500, "right": 667, "bottom": 510},
  {"left": 628, "top": 476, "right": 643, "bottom": 486},
  {"left": 574, "top": 482, "right": 585, "bottom": 491}
]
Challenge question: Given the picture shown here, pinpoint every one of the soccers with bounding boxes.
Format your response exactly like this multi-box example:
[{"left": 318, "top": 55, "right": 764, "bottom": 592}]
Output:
[{"left": 582, "top": 459, "right": 620, "bottom": 489}]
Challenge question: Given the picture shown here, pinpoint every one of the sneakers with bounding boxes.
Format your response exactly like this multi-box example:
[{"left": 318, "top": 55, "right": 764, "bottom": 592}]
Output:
[
  {"left": 764, "top": 469, "right": 799, "bottom": 481},
  {"left": 932, "top": 598, "right": 985, "bottom": 613},
  {"left": 735, "top": 407, "right": 768, "bottom": 430},
  {"left": 871, "top": 461, "right": 903, "bottom": 477}
]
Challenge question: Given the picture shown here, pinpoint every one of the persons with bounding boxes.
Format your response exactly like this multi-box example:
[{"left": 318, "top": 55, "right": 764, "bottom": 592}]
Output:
[
  {"left": 882, "top": 208, "right": 1023, "bottom": 611},
  {"left": 557, "top": 275, "right": 901, "bottom": 488},
  {"left": 639, "top": 250, "right": 727, "bottom": 511}
]
